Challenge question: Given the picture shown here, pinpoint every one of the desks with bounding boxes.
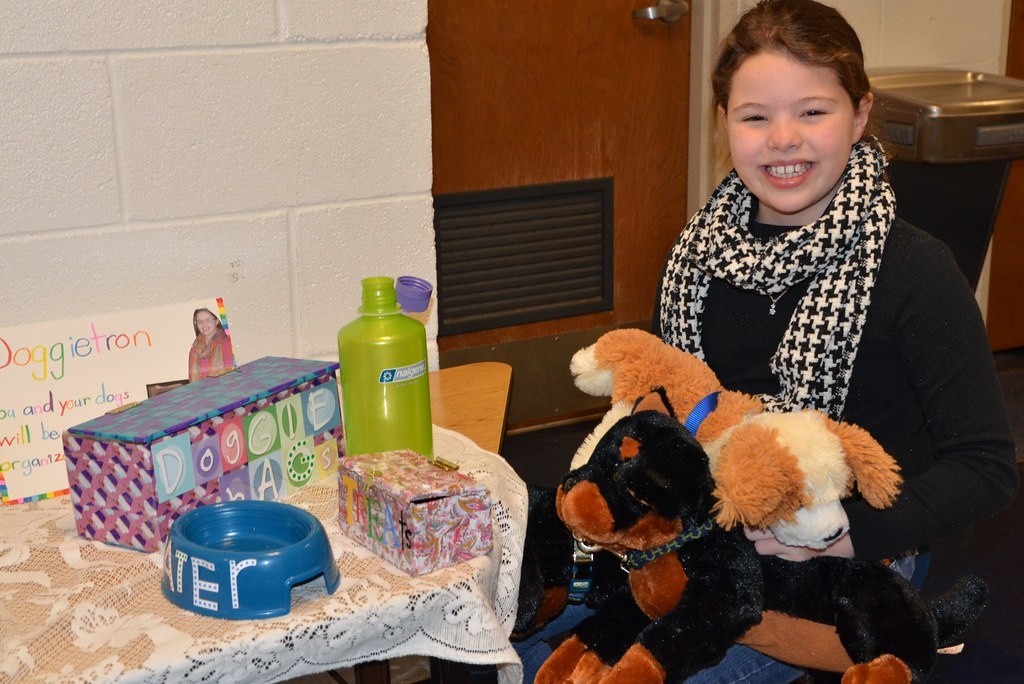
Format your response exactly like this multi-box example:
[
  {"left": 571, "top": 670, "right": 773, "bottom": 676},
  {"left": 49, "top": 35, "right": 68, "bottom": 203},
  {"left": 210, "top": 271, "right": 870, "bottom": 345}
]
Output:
[{"left": 0, "top": 422, "right": 530, "bottom": 684}]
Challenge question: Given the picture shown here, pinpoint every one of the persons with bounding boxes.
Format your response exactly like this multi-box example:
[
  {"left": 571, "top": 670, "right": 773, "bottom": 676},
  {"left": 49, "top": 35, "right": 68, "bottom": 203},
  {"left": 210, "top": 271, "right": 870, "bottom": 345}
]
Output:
[
  {"left": 188, "top": 310, "right": 234, "bottom": 384},
  {"left": 510, "top": 0, "right": 1024, "bottom": 684}
]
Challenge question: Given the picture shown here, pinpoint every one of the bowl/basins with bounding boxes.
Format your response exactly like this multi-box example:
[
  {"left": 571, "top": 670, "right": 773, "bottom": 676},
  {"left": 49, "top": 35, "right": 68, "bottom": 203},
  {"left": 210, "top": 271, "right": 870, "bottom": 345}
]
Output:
[{"left": 161, "top": 501, "right": 340, "bottom": 618}]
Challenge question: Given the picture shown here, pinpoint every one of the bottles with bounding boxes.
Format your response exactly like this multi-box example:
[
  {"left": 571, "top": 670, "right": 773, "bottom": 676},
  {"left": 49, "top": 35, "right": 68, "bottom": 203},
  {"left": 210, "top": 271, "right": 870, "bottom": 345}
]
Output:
[{"left": 337, "top": 276, "right": 433, "bottom": 463}]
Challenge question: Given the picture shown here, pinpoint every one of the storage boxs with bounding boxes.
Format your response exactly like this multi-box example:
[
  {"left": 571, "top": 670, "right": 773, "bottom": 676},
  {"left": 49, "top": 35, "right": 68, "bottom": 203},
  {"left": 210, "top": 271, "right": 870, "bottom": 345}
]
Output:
[
  {"left": 337, "top": 447, "right": 494, "bottom": 576},
  {"left": 61, "top": 355, "right": 340, "bottom": 553}
]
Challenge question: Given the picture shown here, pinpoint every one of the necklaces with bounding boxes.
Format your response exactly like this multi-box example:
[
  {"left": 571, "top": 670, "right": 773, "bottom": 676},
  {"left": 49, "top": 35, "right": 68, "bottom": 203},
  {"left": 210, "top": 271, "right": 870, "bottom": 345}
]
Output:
[{"left": 768, "top": 289, "right": 789, "bottom": 315}]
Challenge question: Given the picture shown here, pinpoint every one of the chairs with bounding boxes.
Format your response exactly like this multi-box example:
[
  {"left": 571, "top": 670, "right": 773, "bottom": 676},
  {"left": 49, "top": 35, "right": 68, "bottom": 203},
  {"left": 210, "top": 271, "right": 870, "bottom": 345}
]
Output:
[
  {"left": 428, "top": 362, "right": 514, "bottom": 455},
  {"left": 430, "top": 157, "right": 1007, "bottom": 683}
]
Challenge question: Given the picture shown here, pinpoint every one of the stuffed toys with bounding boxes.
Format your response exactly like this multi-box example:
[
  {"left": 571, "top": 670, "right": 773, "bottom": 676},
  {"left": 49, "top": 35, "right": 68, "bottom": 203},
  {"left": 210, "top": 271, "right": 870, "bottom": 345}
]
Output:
[
  {"left": 571, "top": 328, "right": 905, "bottom": 551},
  {"left": 536, "top": 409, "right": 988, "bottom": 684}
]
